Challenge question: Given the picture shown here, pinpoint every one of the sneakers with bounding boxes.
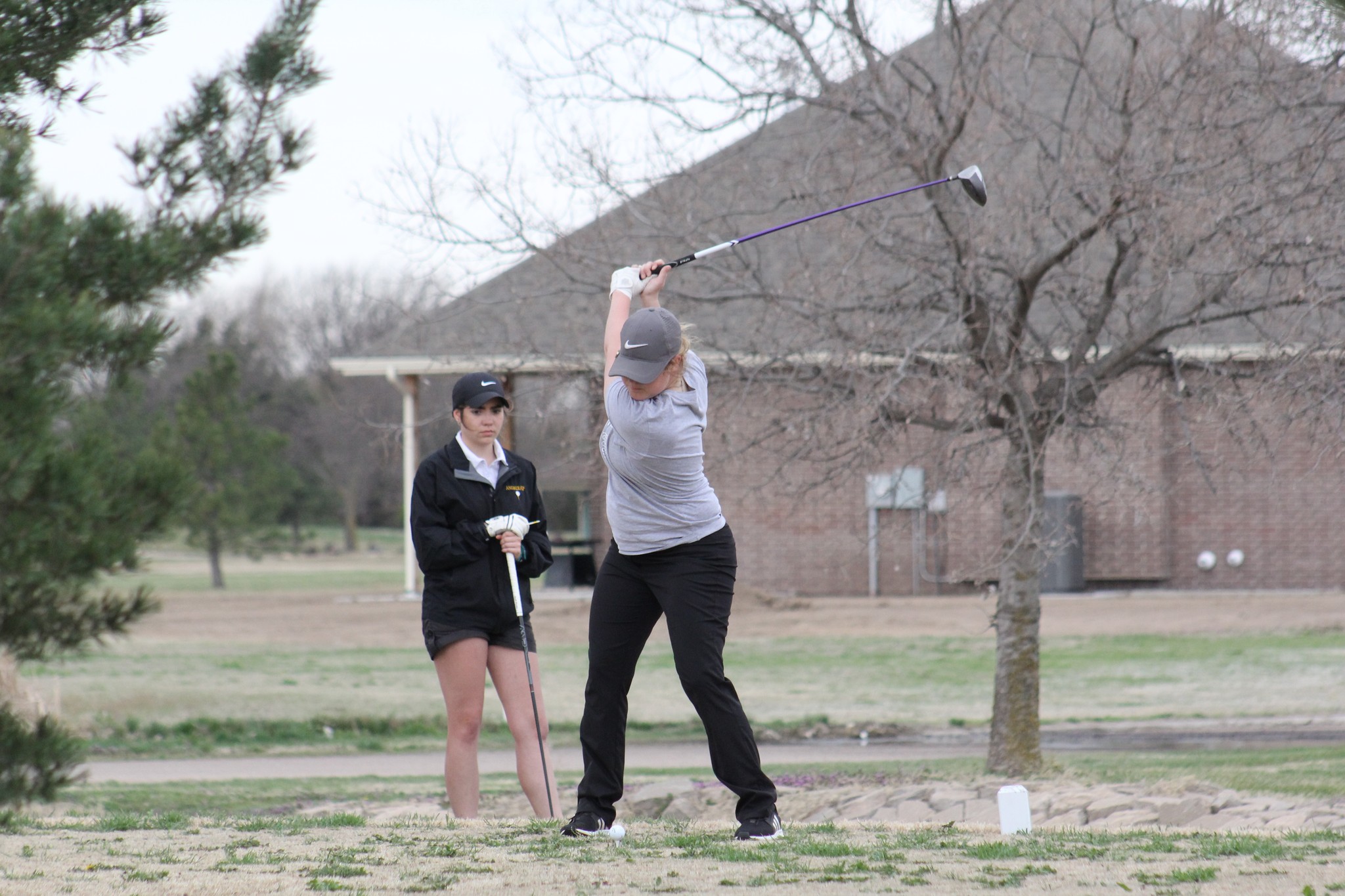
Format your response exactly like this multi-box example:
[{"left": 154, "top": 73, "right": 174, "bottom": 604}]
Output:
[
  {"left": 734, "top": 813, "right": 784, "bottom": 839},
  {"left": 560, "top": 813, "right": 609, "bottom": 838}
]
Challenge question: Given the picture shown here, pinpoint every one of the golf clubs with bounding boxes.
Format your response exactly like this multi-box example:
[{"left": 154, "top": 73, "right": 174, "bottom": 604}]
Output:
[
  {"left": 638, "top": 164, "right": 988, "bottom": 282},
  {"left": 506, "top": 550, "right": 555, "bottom": 818}
]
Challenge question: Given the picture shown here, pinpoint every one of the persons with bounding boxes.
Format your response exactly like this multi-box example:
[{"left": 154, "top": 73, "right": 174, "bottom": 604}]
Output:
[
  {"left": 560, "top": 260, "right": 785, "bottom": 839},
  {"left": 411, "top": 374, "right": 561, "bottom": 821}
]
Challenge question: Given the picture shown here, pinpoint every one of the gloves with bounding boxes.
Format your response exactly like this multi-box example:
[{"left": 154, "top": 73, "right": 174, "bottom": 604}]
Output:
[{"left": 609, "top": 266, "right": 657, "bottom": 301}]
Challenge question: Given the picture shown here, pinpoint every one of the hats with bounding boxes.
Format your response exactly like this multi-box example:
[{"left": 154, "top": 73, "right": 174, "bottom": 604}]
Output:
[
  {"left": 608, "top": 307, "right": 681, "bottom": 383},
  {"left": 452, "top": 373, "right": 510, "bottom": 410}
]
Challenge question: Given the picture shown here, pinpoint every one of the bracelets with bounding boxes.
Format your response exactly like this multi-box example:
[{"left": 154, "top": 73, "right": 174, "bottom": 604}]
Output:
[{"left": 517, "top": 545, "right": 526, "bottom": 563}]
